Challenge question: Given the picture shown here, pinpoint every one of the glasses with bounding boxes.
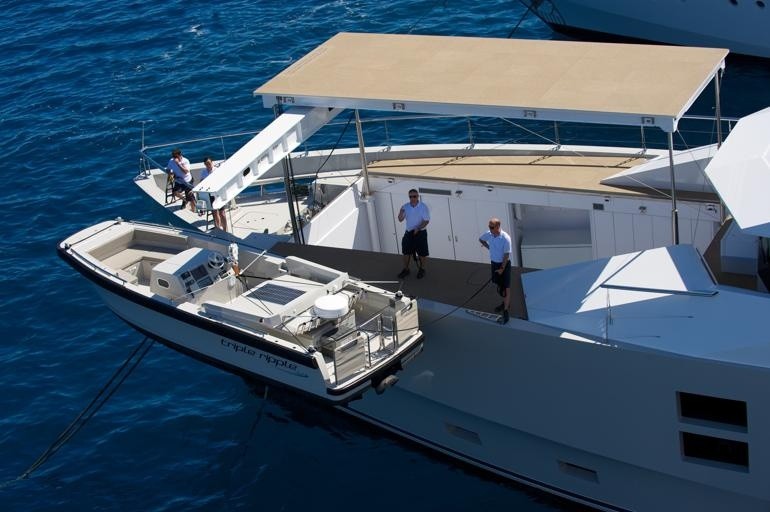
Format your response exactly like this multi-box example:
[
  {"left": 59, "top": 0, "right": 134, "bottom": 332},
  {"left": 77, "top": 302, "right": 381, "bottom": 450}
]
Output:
[
  {"left": 409, "top": 195, "right": 417, "bottom": 198},
  {"left": 489, "top": 225, "right": 497, "bottom": 229}
]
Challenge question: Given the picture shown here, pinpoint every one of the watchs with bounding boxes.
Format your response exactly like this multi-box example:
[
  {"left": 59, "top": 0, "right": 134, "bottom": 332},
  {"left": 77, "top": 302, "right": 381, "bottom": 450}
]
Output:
[{"left": 501, "top": 267, "right": 504, "bottom": 270}]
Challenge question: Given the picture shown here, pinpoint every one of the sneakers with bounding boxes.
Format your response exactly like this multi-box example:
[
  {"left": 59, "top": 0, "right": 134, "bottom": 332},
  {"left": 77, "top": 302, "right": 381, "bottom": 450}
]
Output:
[
  {"left": 181, "top": 198, "right": 188, "bottom": 209},
  {"left": 417, "top": 269, "right": 425, "bottom": 278},
  {"left": 499, "top": 311, "right": 510, "bottom": 324},
  {"left": 495, "top": 302, "right": 509, "bottom": 312},
  {"left": 400, "top": 268, "right": 409, "bottom": 276}
]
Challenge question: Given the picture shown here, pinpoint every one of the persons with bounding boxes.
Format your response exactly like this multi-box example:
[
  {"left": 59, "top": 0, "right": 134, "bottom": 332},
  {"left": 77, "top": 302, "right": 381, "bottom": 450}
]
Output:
[
  {"left": 166, "top": 147, "right": 195, "bottom": 213},
  {"left": 398, "top": 189, "right": 430, "bottom": 279},
  {"left": 200, "top": 157, "right": 227, "bottom": 232},
  {"left": 478, "top": 218, "right": 512, "bottom": 325}
]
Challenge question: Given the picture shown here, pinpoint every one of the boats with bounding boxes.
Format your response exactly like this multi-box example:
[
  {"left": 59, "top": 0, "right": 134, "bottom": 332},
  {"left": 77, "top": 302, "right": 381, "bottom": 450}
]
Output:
[
  {"left": 520, "top": 0, "right": 770, "bottom": 66},
  {"left": 55, "top": 213, "right": 427, "bottom": 409},
  {"left": 53, "top": 29, "right": 770, "bottom": 512}
]
